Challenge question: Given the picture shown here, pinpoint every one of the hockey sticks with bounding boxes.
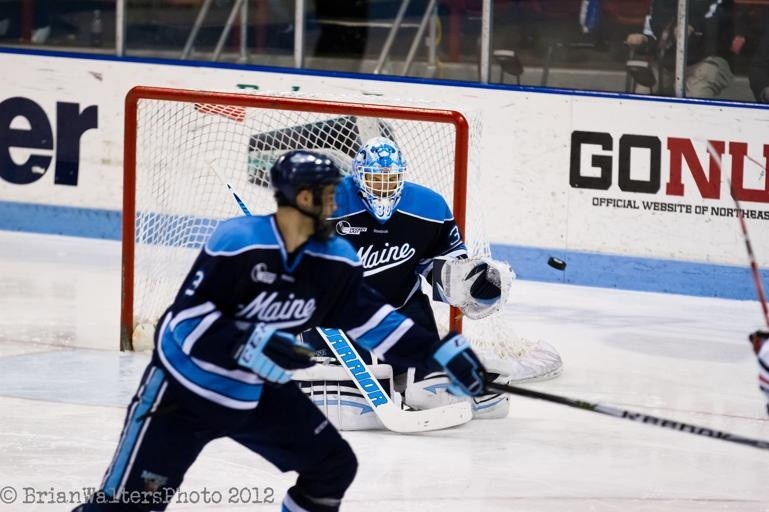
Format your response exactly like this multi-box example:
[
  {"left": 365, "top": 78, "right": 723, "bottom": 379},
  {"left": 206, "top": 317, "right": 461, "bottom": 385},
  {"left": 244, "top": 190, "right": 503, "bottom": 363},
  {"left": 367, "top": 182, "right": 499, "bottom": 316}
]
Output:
[
  {"left": 210, "top": 160, "right": 475, "bottom": 433},
  {"left": 693, "top": 133, "right": 769, "bottom": 328}
]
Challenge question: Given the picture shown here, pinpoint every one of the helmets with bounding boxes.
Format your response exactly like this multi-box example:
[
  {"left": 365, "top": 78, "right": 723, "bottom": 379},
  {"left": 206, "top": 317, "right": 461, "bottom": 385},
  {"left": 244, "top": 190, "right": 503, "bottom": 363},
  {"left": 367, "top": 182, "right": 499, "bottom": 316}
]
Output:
[
  {"left": 271, "top": 151, "right": 338, "bottom": 185},
  {"left": 353, "top": 137, "right": 408, "bottom": 222}
]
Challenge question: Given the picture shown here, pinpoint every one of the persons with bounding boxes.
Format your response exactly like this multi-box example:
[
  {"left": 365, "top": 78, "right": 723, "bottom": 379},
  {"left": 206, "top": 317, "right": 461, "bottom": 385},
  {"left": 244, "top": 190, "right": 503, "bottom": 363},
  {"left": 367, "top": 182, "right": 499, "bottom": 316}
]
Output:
[
  {"left": 73, "top": 149, "right": 489, "bottom": 512},
  {"left": 624, "top": 0, "right": 769, "bottom": 105},
  {"left": 293, "top": 139, "right": 515, "bottom": 432}
]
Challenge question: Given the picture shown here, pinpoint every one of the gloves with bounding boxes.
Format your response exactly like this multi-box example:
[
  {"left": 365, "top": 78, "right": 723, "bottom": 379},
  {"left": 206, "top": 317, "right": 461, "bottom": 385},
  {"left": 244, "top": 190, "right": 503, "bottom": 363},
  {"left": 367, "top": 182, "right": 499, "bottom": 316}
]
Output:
[
  {"left": 432, "top": 330, "right": 488, "bottom": 396},
  {"left": 236, "top": 324, "right": 317, "bottom": 384}
]
